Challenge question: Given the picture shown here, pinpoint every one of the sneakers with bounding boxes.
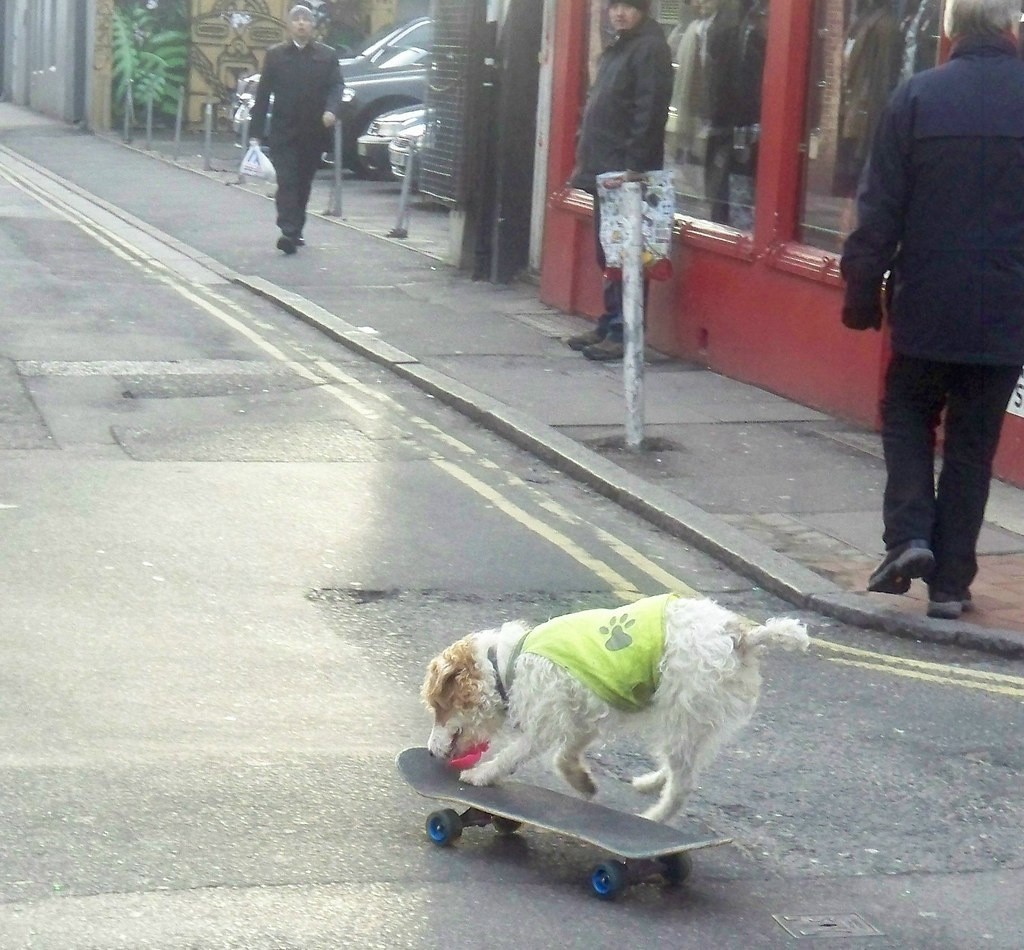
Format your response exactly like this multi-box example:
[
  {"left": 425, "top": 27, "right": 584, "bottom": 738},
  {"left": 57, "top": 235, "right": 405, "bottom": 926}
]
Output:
[
  {"left": 867, "top": 541, "right": 935, "bottom": 595},
  {"left": 927, "top": 580, "right": 972, "bottom": 619},
  {"left": 582, "top": 331, "right": 625, "bottom": 360},
  {"left": 568, "top": 315, "right": 607, "bottom": 351}
]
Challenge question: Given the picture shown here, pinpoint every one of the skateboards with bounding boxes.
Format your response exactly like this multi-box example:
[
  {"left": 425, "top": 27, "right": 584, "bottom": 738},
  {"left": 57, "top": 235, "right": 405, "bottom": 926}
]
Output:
[{"left": 394, "top": 747, "right": 735, "bottom": 902}]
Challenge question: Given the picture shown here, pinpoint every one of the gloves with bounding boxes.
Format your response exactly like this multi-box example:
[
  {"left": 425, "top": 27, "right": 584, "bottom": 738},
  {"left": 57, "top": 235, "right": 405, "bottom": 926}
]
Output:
[{"left": 842, "top": 306, "right": 882, "bottom": 332}]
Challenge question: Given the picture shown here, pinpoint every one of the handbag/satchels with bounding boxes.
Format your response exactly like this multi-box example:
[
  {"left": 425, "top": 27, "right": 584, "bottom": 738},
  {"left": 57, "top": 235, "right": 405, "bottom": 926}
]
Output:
[
  {"left": 239, "top": 138, "right": 278, "bottom": 198},
  {"left": 597, "top": 171, "right": 674, "bottom": 281}
]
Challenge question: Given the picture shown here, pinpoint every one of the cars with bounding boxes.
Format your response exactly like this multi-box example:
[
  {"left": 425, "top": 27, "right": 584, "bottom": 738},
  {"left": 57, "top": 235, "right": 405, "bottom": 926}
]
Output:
[{"left": 232, "top": 17, "right": 436, "bottom": 179}]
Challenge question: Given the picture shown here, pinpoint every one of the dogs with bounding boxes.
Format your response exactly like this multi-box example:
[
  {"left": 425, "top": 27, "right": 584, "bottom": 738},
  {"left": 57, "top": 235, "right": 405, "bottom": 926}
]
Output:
[{"left": 421, "top": 593, "right": 811, "bottom": 825}]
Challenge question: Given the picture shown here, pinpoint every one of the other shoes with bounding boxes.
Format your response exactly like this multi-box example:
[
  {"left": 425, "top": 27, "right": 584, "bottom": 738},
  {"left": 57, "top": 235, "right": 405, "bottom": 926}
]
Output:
[
  {"left": 277, "top": 235, "right": 296, "bottom": 252},
  {"left": 295, "top": 234, "right": 306, "bottom": 246}
]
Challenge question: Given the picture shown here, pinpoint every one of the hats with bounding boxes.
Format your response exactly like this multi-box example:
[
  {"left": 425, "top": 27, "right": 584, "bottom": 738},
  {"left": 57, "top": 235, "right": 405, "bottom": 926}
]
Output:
[
  {"left": 287, "top": 4, "right": 314, "bottom": 27},
  {"left": 609, "top": 0, "right": 647, "bottom": 11}
]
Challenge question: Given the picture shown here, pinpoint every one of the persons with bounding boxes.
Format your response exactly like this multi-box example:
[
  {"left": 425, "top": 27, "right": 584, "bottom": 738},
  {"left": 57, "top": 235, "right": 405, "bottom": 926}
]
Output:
[
  {"left": 569, "top": 0, "right": 675, "bottom": 359},
  {"left": 838, "top": 0, "right": 1024, "bottom": 620},
  {"left": 249, "top": 5, "right": 345, "bottom": 254}
]
